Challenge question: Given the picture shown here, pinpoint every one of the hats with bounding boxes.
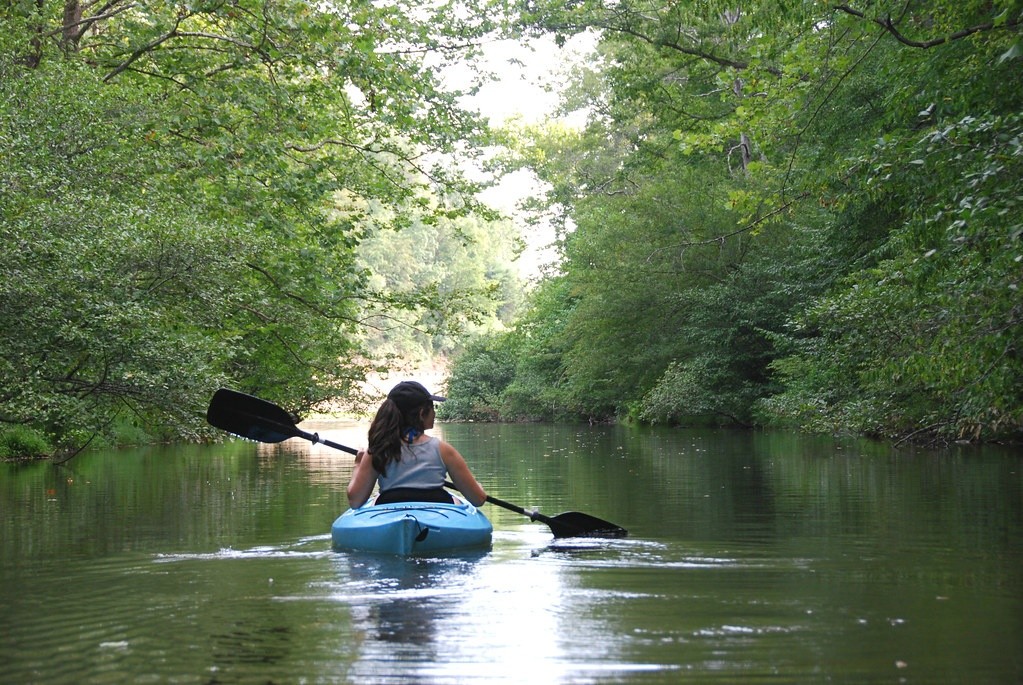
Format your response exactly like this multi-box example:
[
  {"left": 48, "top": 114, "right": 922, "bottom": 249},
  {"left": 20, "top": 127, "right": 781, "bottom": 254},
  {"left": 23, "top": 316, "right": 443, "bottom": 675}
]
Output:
[{"left": 387, "top": 381, "right": 447, "bottom": 404}]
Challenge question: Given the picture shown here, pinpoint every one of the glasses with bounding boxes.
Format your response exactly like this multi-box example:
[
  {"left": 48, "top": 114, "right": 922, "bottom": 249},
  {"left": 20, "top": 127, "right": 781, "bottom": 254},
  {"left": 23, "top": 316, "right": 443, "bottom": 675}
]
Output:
[{"left": 425, "top": 405, "right": 439, "bottom": 410}]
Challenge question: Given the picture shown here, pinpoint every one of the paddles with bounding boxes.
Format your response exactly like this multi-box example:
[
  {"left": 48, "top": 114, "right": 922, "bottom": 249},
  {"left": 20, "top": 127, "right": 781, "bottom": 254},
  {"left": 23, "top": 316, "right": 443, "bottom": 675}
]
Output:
[{"left": 202, "top": 385, "right": 631, "bottom": 542}]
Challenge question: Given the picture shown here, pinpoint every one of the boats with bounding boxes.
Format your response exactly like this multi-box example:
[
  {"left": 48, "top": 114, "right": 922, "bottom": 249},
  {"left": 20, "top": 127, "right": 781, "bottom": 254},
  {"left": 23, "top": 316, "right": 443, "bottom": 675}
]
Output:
[{"left": 329, "top": 494, "right": 491, "bottom": 557}]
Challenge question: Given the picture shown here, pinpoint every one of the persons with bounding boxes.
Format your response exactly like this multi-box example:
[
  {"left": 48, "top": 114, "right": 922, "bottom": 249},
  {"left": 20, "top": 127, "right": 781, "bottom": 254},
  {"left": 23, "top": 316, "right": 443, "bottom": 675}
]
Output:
[{"left": 346, "top": 380, "right": 486, "bottom": 510}]
